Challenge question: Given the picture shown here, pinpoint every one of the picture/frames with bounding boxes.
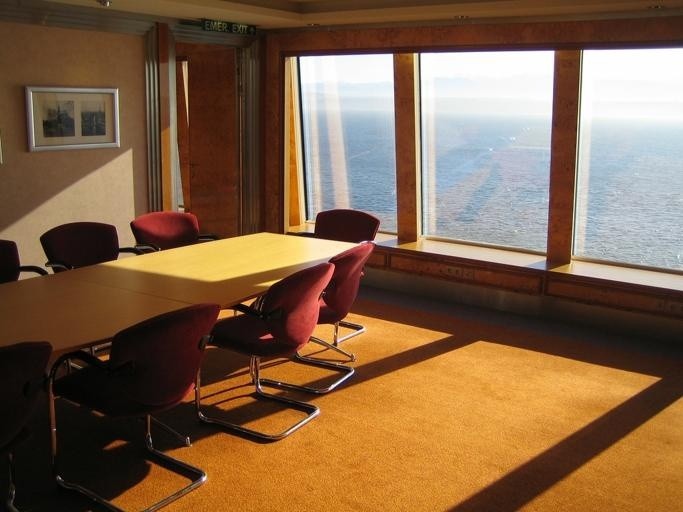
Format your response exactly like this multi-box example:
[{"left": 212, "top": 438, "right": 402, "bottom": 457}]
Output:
[{"left": 24, "top": 85, "right": 121, "bottom": 153}]
